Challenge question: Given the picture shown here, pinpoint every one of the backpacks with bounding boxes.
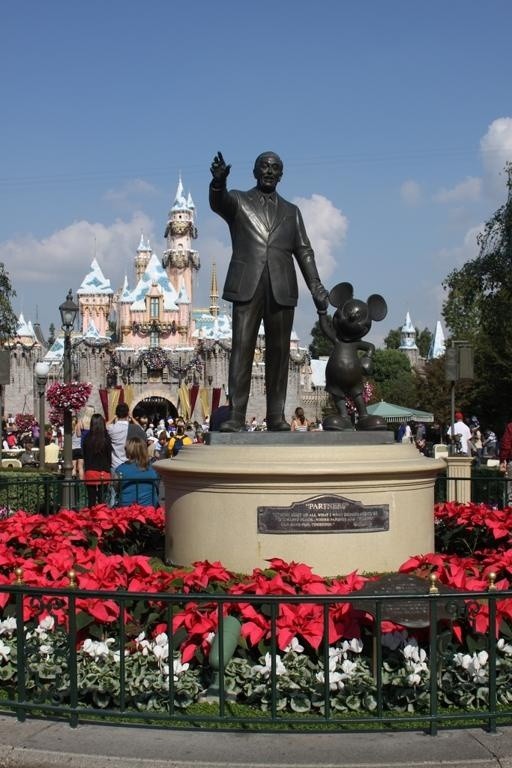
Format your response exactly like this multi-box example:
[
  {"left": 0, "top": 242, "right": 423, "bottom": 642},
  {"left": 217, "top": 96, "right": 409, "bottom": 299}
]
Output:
[{"left": 173, "top": 435, "right": 188, "bottom": 456}]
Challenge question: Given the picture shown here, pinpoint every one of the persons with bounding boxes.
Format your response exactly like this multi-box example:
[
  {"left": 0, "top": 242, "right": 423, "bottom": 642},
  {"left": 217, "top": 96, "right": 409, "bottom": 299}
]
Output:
[
  {"left": 1, "top": 403, "right": 209, "bottom": 508},
  {"left": 399, "top": 411, "right": 512, "bottom": 508},
  {"left": 246, "top": 405, "right": 323, "bottom": 432},
  {"left": 207, "top": 150, "right": 330, "bottom": 433}
]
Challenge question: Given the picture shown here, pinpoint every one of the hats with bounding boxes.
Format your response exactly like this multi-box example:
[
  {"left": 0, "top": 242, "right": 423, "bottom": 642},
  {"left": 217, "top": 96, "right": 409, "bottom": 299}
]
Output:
[{"left": 455, "top": 412, "right": 463, "bottom": 420}]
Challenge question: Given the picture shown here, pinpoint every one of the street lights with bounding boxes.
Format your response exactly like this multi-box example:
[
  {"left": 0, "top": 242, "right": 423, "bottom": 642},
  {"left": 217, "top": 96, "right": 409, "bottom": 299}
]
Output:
[
  {"left": 57, "top": 286, "right": 81, "bottom": 513},
  {"left": 32, "top": 360, "right": 52, "bottom": 472}
]
